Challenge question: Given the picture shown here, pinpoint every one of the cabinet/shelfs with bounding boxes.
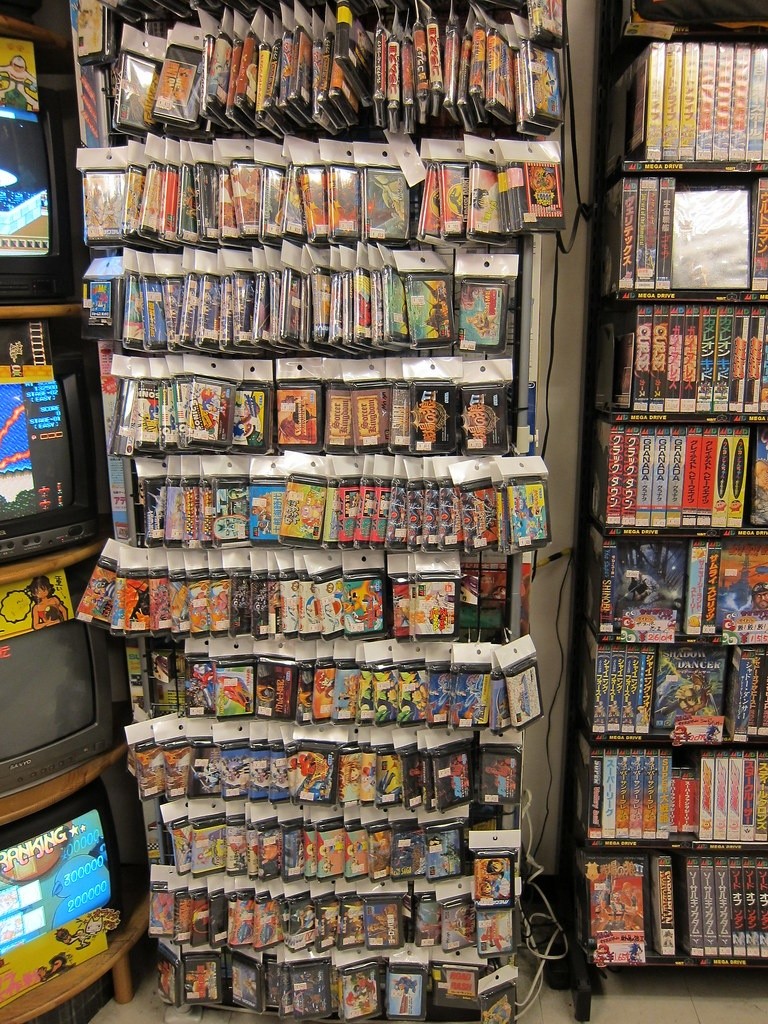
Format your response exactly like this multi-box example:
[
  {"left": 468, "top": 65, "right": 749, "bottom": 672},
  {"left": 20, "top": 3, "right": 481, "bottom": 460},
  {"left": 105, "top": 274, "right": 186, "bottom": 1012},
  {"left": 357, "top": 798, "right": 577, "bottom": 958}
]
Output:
[
  {"left": 0, "top": 14, "right": 156, "bottom": 1023},
  {"left": 554, "top": 1, "right": 768, "bottom": 1021}
]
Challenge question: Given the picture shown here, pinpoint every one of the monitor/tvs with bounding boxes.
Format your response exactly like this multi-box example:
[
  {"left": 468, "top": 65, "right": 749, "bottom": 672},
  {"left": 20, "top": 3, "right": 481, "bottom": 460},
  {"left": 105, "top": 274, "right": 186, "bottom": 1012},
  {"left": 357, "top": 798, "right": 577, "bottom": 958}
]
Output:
[
  {"left": 0, "top": 347, "right": 101, "bottom": 564},
  {"left": 0, "top": 568, "right": 116, "bottom": 799},
  {"left": 0, "top": 105, "right": 75, "bottom": 306},
  {"left": 0, "top": 777, "right": 124, "bottom": 936}
]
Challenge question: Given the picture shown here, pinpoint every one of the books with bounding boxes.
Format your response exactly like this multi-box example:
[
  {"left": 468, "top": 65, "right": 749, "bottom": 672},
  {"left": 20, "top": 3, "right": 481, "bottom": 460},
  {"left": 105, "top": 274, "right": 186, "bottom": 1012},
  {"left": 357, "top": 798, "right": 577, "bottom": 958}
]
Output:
[{"left": 572, "top": 38, "right": 768, "bottom": 958}]
